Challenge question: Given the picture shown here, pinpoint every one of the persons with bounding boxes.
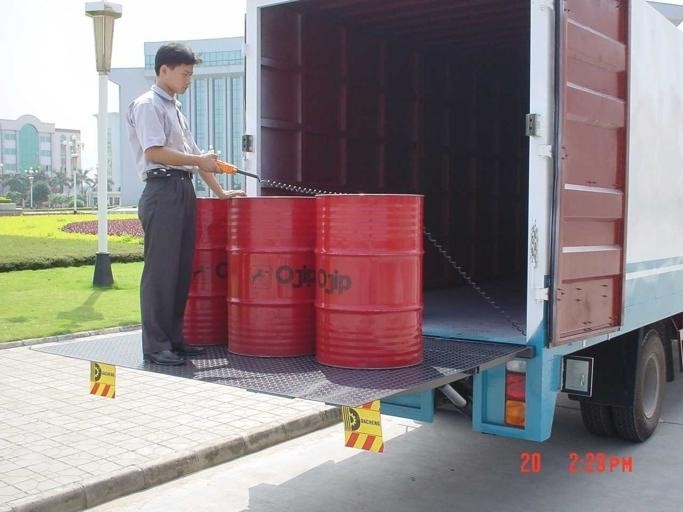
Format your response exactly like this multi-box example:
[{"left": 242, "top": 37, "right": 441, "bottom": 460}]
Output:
[{"left": 123, "top": 41, "right": 246, "bottom": 368}]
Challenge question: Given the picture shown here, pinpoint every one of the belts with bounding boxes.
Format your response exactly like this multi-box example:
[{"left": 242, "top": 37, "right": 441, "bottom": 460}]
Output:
[{"left": 146, "top": 168, "right": 194, "bottom": 180}]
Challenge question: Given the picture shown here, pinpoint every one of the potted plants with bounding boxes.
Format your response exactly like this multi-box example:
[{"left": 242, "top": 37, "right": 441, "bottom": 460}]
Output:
[{"left": 0, "top": 198, "right": 22, "bottom": 216}]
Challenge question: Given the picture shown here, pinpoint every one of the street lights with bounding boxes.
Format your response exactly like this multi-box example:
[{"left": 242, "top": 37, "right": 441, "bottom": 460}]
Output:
[
  {"left": 82, "top": 0, "right": 126, "bottom": 288},
  {"left": 0, "top": 162, "right": 4, "bottom": 194},
  {"left": 61, "top": 133, "right": 86, "bottom": 213},
  {"left": 23, "top": 166, "right": 38, "bottom": 211},
  {"left": 201, "top": 144, "right": 223, "bottom": 198}
]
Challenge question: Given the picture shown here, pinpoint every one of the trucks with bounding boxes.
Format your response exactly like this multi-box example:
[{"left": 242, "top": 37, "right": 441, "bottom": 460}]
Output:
[{"left": 32, "top": 0, "right": 683, "bottom": 451}]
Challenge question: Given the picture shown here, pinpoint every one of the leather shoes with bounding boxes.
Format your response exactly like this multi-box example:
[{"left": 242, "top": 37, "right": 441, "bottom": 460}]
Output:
[
  {"left": 172, "top": 342, "right": 206, "bottom": 356},
  {"left": 143, "top": 350, "right": 187, "bottom": 366}
]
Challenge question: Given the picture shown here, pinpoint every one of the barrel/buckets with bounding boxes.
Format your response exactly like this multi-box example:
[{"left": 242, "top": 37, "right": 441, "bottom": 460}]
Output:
[
  {"left": 226, "top": 195, "right": 315, "bottom": 357},
  {"left": 314, "top": 193, "right": 425, "bottom": 370},
  {"left": 183, "top": 197, "right": 226, "bottom": 347}
]
